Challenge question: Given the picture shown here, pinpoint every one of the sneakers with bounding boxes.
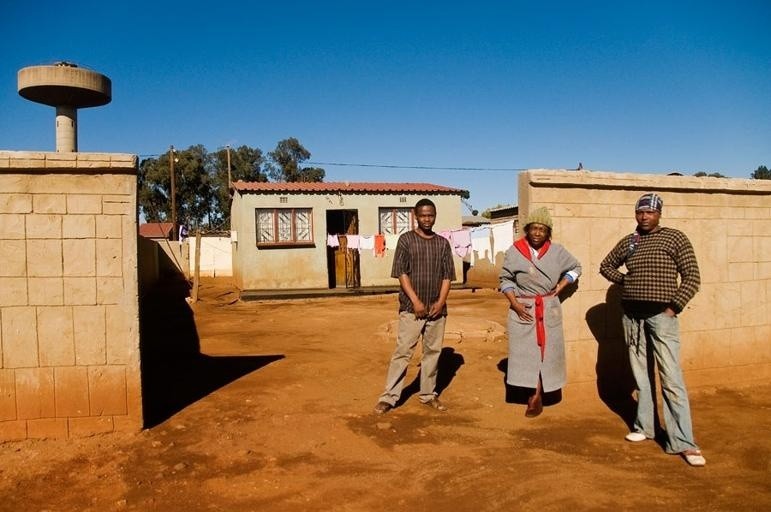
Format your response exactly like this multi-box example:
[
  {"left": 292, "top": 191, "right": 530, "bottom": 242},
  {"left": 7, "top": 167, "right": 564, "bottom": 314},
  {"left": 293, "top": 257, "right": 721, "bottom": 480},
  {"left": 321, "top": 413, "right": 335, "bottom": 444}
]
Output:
[
  {"left": 426, "top": 395, "right": 449, "bottom": 411},
  {"left": 372, "top": 400, "right": 392, "bottom": 415},
  {"left": 682, "top": 449, "right": 708, "bottom": 465},
  {"left": 624, "top": 430, "right": 648, "bottom": 442}
]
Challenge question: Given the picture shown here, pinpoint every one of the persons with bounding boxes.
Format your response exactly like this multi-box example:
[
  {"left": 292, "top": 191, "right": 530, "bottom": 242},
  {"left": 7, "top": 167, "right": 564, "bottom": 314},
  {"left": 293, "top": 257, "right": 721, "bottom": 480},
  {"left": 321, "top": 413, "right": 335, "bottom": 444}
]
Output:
[
  {"left": 372, "top": 198, "right": 456, "bottom": 414},
  {"left": 498, "top": 206, "right": 583, "bottom": 418},
  {"left": 600, "top": 193, "right": 706, "bottom": 467}
]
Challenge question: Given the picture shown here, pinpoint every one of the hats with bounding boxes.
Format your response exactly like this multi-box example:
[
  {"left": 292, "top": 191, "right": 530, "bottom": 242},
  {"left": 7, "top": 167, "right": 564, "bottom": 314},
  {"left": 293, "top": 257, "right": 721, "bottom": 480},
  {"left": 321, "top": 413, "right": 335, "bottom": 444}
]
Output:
[{"left": 522, "top": 206, "right": 554, "bottom": 235}]
